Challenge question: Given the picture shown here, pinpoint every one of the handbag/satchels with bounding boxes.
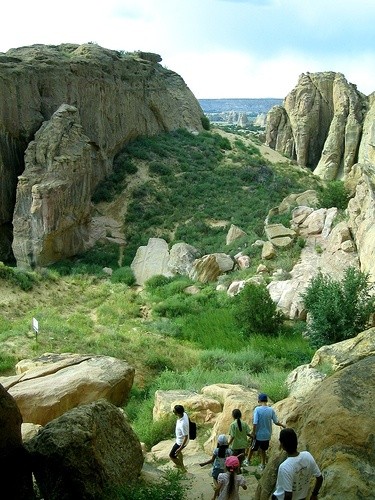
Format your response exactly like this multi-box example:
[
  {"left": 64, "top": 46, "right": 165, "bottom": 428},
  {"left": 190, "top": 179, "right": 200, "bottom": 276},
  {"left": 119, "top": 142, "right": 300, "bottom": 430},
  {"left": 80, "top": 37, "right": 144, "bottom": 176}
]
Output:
[{"left": 212, "top": 468, "right": 224, "bottom": 480}]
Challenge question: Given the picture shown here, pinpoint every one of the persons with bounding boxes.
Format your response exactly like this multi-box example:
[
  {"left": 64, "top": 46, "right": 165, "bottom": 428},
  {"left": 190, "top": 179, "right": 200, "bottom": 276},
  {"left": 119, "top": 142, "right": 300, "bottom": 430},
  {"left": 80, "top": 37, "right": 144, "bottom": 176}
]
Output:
[
  {"left": 199, "top": 435, "right": 248, "bottom": 498},
  {"left": 228, "top": 409, "right": 253, "bottom": 474},
  {"left": 271, "top": 428, "right": 323, "bottom": 500},
  {"left": 243, "top": 393, "right": 286, "bottom": 471},
  {"left": 212, "top": 456, "right": 247, "bottom": 500},
  {"left": 169, "top": 405, "right": 189, "bottom": 473}
]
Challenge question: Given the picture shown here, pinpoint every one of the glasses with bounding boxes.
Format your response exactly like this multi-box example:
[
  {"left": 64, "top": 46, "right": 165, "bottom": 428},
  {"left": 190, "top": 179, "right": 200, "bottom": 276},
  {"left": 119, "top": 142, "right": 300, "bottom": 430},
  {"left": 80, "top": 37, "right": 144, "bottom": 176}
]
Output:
[{"left": 173, "top": 410, "right": 177, "bottom": 414}]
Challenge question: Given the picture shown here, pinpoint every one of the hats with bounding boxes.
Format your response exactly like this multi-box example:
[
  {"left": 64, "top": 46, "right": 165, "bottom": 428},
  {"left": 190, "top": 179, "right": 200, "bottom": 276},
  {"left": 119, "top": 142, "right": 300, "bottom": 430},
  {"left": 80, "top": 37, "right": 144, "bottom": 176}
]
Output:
[
  {"left": 258, "top": 394, "right": 268, "bottom": 402},
  {"left": 225, "top": 456, "right": 240, "bottom": 466},
  {"left": 218, "top": 435, "right": 227, "bottom": 444},
  {"left": 279, "top": 428, "right": 298, "bottom": 446}
]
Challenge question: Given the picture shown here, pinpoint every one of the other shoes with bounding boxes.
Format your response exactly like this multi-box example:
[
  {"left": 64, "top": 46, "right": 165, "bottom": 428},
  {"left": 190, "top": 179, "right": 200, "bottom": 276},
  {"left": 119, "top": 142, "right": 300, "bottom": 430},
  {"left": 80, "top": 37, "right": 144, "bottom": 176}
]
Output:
[
  {"left": 259, "top": 464, "right": 265, "bottom": 470},
  {"left": 243, "top": 458, "right": 249, "bottom": 465}
]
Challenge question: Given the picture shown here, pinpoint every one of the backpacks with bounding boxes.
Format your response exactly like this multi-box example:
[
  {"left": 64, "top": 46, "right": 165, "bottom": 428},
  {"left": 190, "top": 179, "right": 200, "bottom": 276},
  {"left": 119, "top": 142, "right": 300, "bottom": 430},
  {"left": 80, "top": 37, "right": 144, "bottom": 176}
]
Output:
[{"left": 185, "top": 412, "right": 197, "bottom": 440}]
